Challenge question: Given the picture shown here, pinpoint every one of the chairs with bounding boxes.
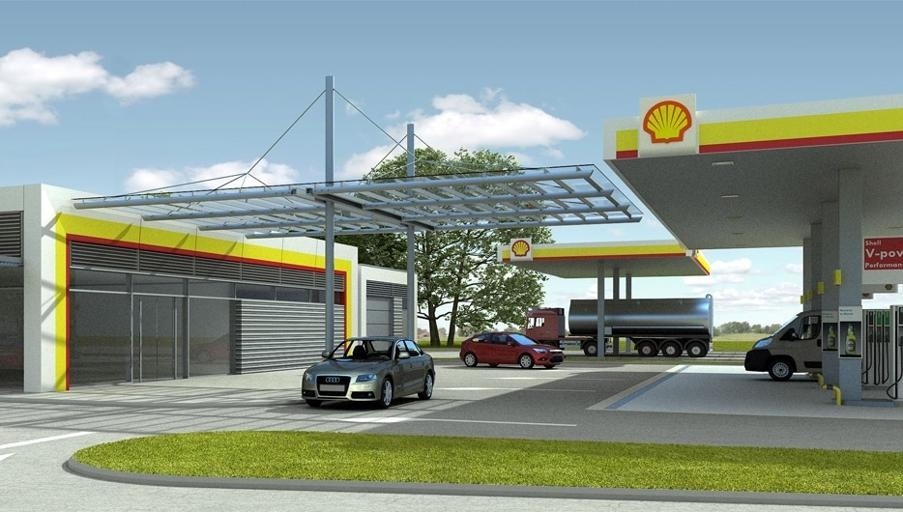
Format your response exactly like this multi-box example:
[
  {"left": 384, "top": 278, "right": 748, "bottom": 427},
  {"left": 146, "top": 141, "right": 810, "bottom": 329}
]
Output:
[
  {"left": 352, "top": 345, "right": 364, "bottom": 356},
  {"left": 495, "top": 337, "right": 499, "bottom": 341}
]
